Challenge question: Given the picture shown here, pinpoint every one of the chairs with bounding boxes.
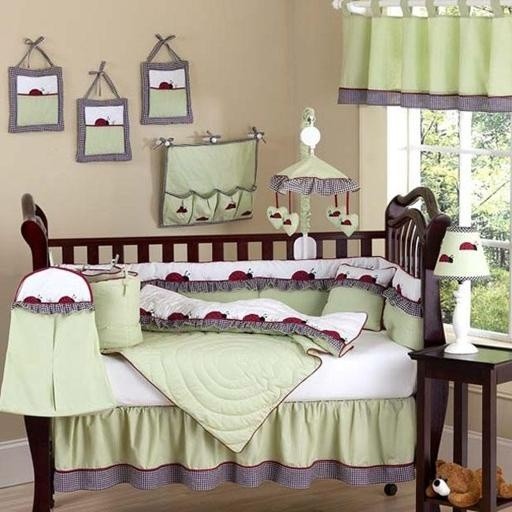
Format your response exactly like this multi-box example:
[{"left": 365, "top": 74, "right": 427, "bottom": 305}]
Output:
[{"left": 0, "top": 187, "right": 451, "bottom": 512}]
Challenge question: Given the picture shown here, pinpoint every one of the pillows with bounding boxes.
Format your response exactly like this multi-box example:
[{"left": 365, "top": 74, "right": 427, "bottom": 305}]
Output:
[
  {"left": 322, "top": 262, "right": 396, "bottom": 332},
  {"left": 79, "top": 255, "right": 143, "bottom": 353}
]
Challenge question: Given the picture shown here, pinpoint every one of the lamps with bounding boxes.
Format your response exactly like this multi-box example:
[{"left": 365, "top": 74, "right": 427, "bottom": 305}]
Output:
[{"left": 434, "top": 227, "right": 491, "bottom": 355}]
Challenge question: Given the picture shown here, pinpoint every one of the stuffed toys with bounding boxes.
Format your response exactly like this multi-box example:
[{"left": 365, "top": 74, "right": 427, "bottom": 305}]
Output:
[{"left": 425, "top": 460, "right": 512, "bottom": 508}]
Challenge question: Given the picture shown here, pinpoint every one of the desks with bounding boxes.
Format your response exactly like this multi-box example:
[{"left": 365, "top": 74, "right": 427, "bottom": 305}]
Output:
[{"left": 408, "top": 343, "right": 511, "bottom": 512}]
